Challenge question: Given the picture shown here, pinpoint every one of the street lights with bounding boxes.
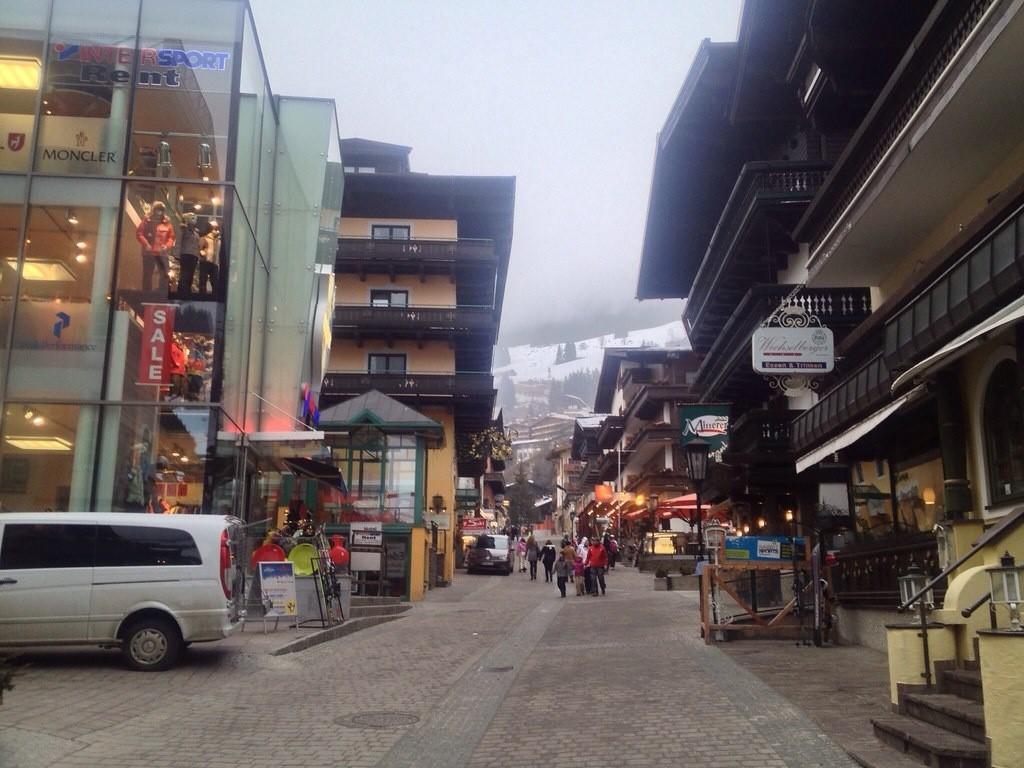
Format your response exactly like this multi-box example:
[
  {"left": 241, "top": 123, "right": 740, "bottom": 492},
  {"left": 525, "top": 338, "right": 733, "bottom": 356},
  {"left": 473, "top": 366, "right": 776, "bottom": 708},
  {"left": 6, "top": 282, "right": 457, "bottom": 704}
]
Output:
[
  {"left": 602, "top": 448, "right": 622, "bottom": 549},
  {"left": 680, "top": 434, "right": 712, "bottom": 562},
  {"left": 563, "top": 393, "right": 590, "bottom": 411}
]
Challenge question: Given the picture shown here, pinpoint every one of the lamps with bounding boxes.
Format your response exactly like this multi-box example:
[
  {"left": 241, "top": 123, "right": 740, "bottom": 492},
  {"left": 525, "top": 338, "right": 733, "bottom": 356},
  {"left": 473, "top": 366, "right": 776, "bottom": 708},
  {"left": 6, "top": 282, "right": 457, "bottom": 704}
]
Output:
[
  {"left": 705, "top": 518, "right": 726, "bottom": 550},
  {"left": 984, "top": 551, "right": 1023, "bottom": 632},
  {"left": 897, "top": 562, "right": 935, "bottom": 624}
]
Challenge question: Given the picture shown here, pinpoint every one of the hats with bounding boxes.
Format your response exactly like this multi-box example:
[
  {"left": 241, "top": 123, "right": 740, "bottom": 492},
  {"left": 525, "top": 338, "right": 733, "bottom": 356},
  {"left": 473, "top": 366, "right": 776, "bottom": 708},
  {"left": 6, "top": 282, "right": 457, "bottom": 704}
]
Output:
[{"left": 610, "top": 535, "right": 614, "bottom": 537}]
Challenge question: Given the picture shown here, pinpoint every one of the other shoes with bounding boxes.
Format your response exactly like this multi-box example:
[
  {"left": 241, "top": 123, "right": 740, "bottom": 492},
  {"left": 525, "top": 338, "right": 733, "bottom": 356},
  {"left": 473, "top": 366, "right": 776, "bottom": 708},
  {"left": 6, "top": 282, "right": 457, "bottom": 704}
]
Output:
[
  {"left": 602, "top": 590, "right": 605, "bottom": 594},
  {"left": 593, "top": 592, "right": 598, "bottom": 596}
]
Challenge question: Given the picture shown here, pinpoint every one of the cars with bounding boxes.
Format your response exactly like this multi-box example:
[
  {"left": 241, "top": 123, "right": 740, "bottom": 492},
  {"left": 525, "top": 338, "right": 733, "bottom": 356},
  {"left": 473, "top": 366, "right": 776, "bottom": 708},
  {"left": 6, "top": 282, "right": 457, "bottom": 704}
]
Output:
[{"left": 466, "top": 534, "right": 515, "bottom": 575}]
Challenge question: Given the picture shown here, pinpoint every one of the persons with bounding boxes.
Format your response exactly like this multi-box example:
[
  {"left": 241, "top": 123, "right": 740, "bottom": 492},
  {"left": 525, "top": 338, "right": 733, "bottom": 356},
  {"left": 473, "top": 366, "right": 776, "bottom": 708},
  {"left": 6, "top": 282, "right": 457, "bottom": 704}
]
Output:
[
  {"left": 261, "top": 506, "right": 320, "bottom": 550},
  {"left": 559, "top": 531, "right": 619, "bottom": 596},
  {"left": 516, "top": 537, "right": 528, "bottom": 572},
  {"left": 524, "top": 534, "right": 541, "bottom": 580},
  {"left": 166, "top": 332, "right": 207, "bottom": 404},
  {"left": 538, "top": 539, "right": 556, "bottom": 581},
  {"left": 134, "top": 200, "right": 221, "bottom": 296},
  {"left": 551, "top": 550, "right": 574, "bottom": 598},
  {"left": 486, "top": 517, "right": 558, "bottom": 542},
  {"left": 119, "top": 435, "right": 165, "bottom": 514}
]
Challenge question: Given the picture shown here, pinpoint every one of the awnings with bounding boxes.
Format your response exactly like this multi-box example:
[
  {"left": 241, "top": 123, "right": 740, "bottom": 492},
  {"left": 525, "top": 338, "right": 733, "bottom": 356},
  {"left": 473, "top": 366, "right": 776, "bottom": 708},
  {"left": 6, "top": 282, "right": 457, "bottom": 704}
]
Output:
[
  {"left": 282, "top": 456, "right": 350, "bottom": 494},
  {"left": 790, "top": 349, "right": 935, "bottom": 474},
  {"left": 881, "top": 204, "right": 1024, "bottom": 393}
]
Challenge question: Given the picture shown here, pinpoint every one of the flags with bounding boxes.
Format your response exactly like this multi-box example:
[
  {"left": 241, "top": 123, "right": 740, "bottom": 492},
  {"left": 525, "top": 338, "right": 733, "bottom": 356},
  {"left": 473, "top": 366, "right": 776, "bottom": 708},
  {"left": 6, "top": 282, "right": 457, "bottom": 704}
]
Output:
[{"left": 679, "top": 404, "right": 734, "bottom": 477}]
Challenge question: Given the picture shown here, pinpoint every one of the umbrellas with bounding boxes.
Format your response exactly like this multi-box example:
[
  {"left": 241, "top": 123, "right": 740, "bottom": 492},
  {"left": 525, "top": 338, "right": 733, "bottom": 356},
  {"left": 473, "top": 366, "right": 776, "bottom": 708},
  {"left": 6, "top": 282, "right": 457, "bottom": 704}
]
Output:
[{"left": 621, "top": 493, "right": 733, "bottom": 543}]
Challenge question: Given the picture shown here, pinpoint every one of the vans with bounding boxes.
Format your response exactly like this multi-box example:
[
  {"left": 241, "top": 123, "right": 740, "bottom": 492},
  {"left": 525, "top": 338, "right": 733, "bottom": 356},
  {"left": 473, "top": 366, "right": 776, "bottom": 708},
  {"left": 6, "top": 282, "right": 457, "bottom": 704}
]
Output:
[{"left": 0, "top": 511, "right": 246, "bottom": 672}]
